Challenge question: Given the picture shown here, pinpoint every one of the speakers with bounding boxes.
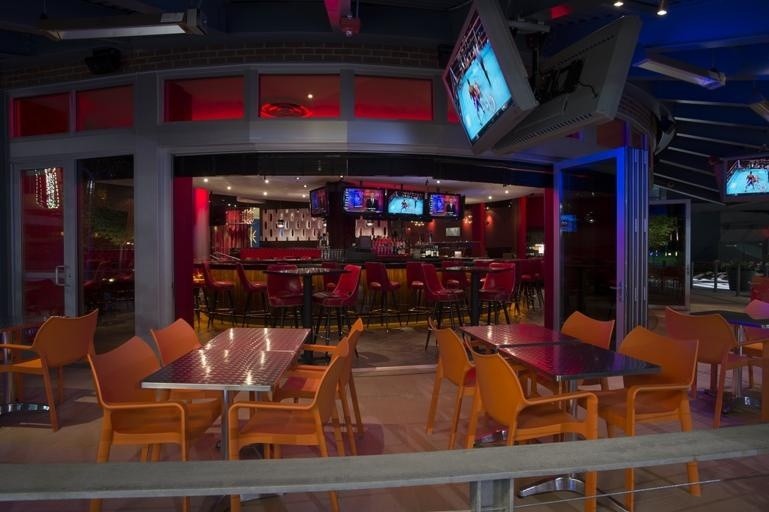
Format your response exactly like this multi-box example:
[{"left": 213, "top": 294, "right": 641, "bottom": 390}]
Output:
[{"left": 210, "top": 205, "right": 225, "bottom": 226}]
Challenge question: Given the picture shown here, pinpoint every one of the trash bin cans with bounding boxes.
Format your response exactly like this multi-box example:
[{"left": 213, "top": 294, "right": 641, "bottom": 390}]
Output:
[{"left": 751, "top": 276, "right": 769, "bottom": 303}]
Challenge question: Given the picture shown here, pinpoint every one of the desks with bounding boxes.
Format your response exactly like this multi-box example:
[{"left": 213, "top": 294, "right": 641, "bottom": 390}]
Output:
[
  {"left": 459, "top": 324, "right": 660, "bottom": 498},
  {"left": 141, "top": 328, "right": 312, "bottom": 503},
  {"left": 689, "top": 311, "right": 769, "bottom": 415}
]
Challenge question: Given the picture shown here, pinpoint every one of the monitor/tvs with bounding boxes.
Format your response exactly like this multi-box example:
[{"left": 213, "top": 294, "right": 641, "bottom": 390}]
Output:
[
  {"left": 426, "top": 192, "right": 462, "bottom": 219},
  {"left": 310, "top": 186, "right": 331, "bottom": 217},
  {"left": 385, "top": 189, "right": 428, "bottom": 221},
  {"left": 491, "top": 15, "right": 642, "bottom": 153},
  {"left": 719, "top": 152, "right": 769, "bottom": 202},
  {"left": 339, "top": 185, "right": 385, "bottom": 216},
  {"left": 441, "top": 0, "right": 539, "bottom": 154}
]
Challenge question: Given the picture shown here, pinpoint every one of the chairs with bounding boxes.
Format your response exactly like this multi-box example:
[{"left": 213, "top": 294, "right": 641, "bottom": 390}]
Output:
[
  {"left": 84, "top": 260, "right": 134, "bottom": 326},
  {"left": 577, "top": 326, "right": 701, "bottom": 511},
  {"left": 464, "top": 336, "right": 599, "bottom": 512},
  {"left": 228, "top": 336, "right": 350, "bottom": 512},
  {"left": 272, "top": 317, "right": 364, "bottom": 459},
  {"left": 663, "top": 305, "right": 769, "bottom": 429},
  {"left": 0, "top": 309, "right": 102, "bottom": 431},
  {"left": 425, "top": 317, "right": 529, "bottom": 450},
  {"left": 87, "top": 335, "right": 223, "bottom": 512},
  {"left": 150, "top": 318, "right": 240, "bottom": 461},
  {"left": 742, "top": 299, "right": 769, "bottom": 389},
  {"left": 528, "top": 311, "right": 616, "bottom": 442}
]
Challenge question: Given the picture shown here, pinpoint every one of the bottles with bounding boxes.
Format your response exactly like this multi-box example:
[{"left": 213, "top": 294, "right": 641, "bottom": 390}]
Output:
[
  {"left": 318, "top": 233, "right": 344, "bottom": 261},
  {"left": 372, "top": 235, "right": 439, "bottom": 258},
  {"left": 667, "top": 230, "right": 682, "bottom": 257}
]
